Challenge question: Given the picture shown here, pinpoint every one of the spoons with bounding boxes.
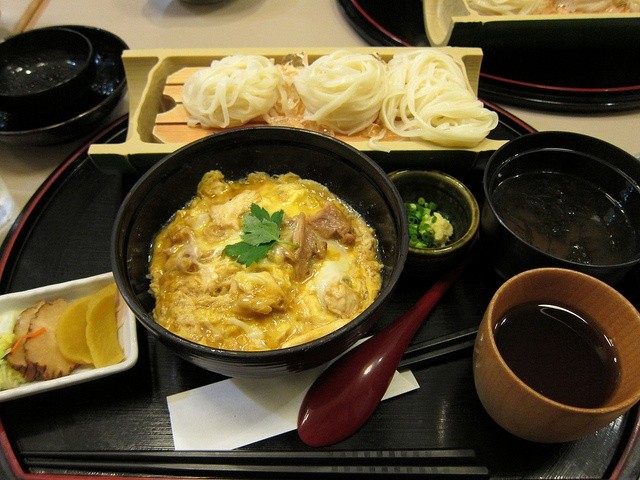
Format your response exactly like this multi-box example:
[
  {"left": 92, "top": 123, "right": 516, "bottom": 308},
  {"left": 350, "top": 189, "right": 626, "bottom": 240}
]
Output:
[{"left": 297, "top": 279, "right": 450, "bottom": 448}]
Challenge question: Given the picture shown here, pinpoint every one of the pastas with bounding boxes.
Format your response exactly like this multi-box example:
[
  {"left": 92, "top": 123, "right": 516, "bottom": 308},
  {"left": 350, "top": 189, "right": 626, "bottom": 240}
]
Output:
[
  {"left": 386, "top": 49, "right": 499, "bottom": 147},
  {"left": 296, "top": 49, "right": 384, "bottom": 137},
  {"left": 184, "top": 54, "right": 278, "bottom": 128}
]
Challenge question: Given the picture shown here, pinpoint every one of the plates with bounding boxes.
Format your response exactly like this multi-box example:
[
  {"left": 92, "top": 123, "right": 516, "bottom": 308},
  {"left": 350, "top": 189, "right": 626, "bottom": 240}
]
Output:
[
  {"left": 0, "top": 270, "right": 138, "bottom": 401},
  {"left": 338, "top": 1, "right": 640, "bottom": 116}
]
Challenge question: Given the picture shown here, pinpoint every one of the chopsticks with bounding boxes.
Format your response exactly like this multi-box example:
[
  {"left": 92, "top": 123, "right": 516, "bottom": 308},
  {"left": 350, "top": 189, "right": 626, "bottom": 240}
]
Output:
[{"left": 396, "top": 326, "right": 479, "bottom": 372}]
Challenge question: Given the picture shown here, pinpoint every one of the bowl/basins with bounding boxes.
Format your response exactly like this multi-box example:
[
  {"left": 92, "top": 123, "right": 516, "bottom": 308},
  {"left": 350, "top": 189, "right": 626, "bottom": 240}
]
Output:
[
  {"left": 0, "top": 25, "right": 130, "bottom": 149},
  {"left": 1, "top": 28, "right": 95, "bottom": 113},
  {"left": 108, "top": 126, "right": 409, "bottom": 380},
  {"left": 472, "top": 266, "right": 640, "bottom": 443},
  {"left": 385, "top": 167, "right": 479, "bottom": 254},
  {"left": 480, "top": 130, "right": 639, "bottom": 273}
]
